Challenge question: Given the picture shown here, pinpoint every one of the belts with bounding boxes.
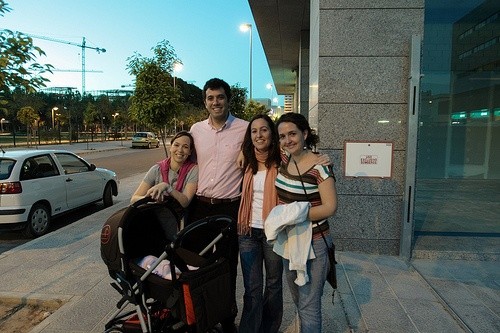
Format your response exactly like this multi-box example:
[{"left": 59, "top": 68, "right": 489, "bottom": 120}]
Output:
[{"left": 196, "top": 195, "right": 238, "bottom": 205}]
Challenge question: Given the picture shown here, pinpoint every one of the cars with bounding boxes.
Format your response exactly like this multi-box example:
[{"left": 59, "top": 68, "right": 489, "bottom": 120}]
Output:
[{"left": 0, "top": 146, "right": 120, "bottom": 237}]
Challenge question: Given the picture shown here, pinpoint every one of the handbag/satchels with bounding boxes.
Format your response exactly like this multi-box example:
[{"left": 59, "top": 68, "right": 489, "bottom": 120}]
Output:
[{"left": 327, "top": 242, "right": 338, "bottom": 290}]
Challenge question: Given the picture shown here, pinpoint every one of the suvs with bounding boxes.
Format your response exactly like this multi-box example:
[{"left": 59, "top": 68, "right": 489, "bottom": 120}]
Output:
[{"left": 131, "top": 132, "right": 160, "bottom": 148}]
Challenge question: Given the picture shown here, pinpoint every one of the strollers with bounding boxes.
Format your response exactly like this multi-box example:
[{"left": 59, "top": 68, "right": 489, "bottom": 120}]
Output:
[{"left": 100, "top": 194, "right": 236, "bottom": 333}]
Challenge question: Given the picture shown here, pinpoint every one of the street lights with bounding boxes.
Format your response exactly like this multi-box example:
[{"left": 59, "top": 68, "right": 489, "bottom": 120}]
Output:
[
  {"left": 239, "top": 23, "right": 252, "bottom": 101},
  {"left": 113, "top": 111, "right": 119, "bottom": 132},
  {"left": 52, "top": 106, "right": 58, "bottom": 140},
  {"left": 267, "top": 83, "right": 273, "bottom": 109},
  {"left": 173, "top": 59, "right": 183, "bottom": 134}
]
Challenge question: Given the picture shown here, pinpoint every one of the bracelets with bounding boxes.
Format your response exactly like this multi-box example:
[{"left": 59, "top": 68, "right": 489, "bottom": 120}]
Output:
[{"left": 167, "top": 187, "right": 173, "bottom": 193}]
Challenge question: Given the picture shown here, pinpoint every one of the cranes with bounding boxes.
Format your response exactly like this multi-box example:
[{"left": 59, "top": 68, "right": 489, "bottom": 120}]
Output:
[{"left": 28, "top": 31, "right": 106, "bottom": 98}]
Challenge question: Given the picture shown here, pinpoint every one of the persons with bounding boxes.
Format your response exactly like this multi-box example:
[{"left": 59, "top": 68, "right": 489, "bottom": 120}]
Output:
[
  {"left": 131, "top": 130, "right": 199, "bottom": 235},
  {"left": 137, "top": 255, "right": 182, "bottom": 280},
  {"left": 237, "top": 114, "right": 333, "bottom": 333},
  {"left": 237, "top": 112, "right": 338, "bottom": 333},
  {"left": 188, "top": 78, "right": 250, "bottom": 333}
]
